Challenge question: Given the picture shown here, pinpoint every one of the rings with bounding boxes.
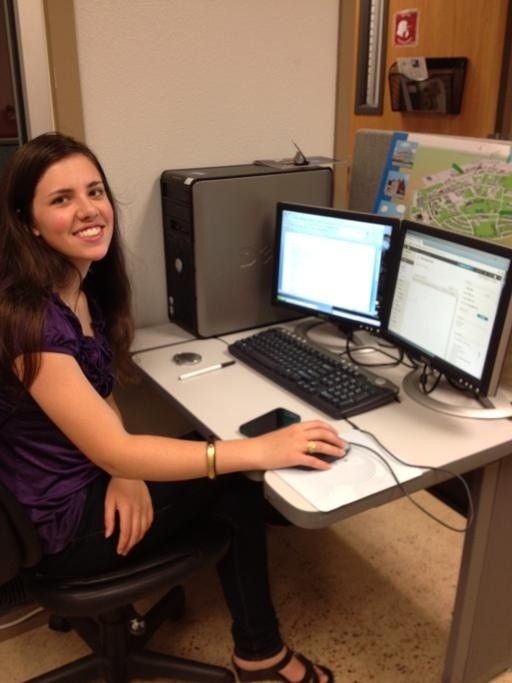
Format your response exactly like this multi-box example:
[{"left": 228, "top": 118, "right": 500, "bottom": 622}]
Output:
[{"left": 306, "top": 440, "right": 316, "bottom": 454}]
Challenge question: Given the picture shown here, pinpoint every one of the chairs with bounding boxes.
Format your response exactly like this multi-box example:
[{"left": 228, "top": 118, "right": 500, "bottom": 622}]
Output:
[{"left": 1, "top": 483, "right": 242, "bottom": 683}]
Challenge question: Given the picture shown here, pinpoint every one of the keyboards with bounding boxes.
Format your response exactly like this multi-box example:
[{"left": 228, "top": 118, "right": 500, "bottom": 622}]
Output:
[{"left": 227, "top": 326, "right": 401, "bottom": 421}]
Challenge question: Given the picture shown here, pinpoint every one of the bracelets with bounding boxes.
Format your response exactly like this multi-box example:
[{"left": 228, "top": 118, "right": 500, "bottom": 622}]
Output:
[{"left": 206, "top": 436, "right": 217, "bottom": 479}]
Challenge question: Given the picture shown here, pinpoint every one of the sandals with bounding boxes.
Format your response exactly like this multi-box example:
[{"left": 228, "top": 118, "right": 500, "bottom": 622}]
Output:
[{"left": 231, "top": 647, "right": 334, "bottom": 683}]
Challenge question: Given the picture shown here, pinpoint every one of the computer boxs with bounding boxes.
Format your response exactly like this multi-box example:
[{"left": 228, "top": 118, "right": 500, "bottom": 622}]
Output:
[{"left": 159, "top": 157, "right": 334, "bottom": 339}]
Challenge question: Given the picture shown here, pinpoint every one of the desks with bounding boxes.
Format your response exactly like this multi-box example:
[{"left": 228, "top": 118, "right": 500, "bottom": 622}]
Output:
[{"left": 130, "top": 319, "right": 512, "bottom": 683}]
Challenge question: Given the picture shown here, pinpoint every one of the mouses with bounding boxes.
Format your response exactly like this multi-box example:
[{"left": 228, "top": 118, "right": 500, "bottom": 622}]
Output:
[{"left": 290, "top": 436, "right": 351, "bottom": 471}]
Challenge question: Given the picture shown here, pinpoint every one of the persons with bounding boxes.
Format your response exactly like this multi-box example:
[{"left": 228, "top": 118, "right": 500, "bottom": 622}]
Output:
[{"left": 1, "top": 130, "right": 347, "bottom": 682}]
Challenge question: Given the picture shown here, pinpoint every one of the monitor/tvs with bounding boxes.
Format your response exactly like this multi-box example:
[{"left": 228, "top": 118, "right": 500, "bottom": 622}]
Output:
[
  {"left": 273, "top": 200, "right": 401, "bottom": 357},
  {"left": 380, "top": 218, "right": 512, "bottom": 420}
]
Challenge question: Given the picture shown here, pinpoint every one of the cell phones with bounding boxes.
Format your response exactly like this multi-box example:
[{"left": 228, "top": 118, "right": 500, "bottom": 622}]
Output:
[{"left": 239, "top": 407, "right": 301, "bottom": 438}]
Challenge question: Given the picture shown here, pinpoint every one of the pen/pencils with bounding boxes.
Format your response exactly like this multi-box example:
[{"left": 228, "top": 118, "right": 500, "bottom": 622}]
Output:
[{"left": 178, "top": 361, "right": 235, "bottom": 380}]
